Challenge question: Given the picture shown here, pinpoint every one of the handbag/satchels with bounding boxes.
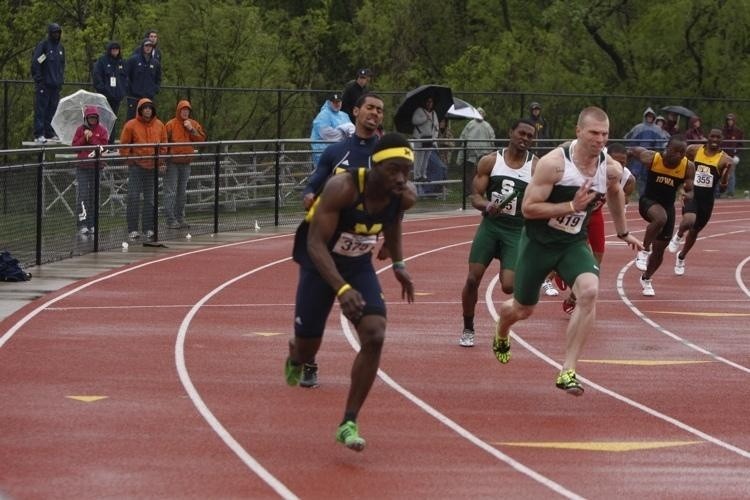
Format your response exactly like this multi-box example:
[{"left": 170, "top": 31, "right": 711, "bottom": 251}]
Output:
[
  {"left": 421, "top": 136, "right": 433, "bottom": 147},
  {"left": 0, "top": 250, "right": 27, "bottom": 280}
]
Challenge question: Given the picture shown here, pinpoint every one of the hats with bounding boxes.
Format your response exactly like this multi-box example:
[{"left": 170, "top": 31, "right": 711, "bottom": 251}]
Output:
[
  {"left": 330, "top": 94, "right": 342, "bottom": 102},
  {"left": 87, "top": 114, "right": 98, "bottom": 117},
  {"left": 532, "top": 105, "right": 541, "bottom": 110},
  {"left": 358, "top": 70, "right": 368, "bottom": 78},
  {"left": 140, "top": 102, "right": 154, "bottom": 110}
]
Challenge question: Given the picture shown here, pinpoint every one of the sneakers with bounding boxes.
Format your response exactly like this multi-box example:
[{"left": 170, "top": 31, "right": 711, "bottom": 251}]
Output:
[
  {"left": 335, "top": 419, "right": 366, "bottom": 452},
  {"left": 541, "top": 279, "right": 559, "bottom": 296},
  {"left": 165, "top": 220, "right": 190, "bottom": 229},
  {"left": 674, "top": 251, "right": 685, "bottom": 275},
  {"left": 556, "top": 368, "right": 584, "bottom": 396},
  {"left": 460, "top": 327, "right": 475, "bottom": 347},
  {"left": 80, "top": 226, "right": 95, "bottom": 233},
  {"left": 668, "top": 230, "right": 685, "bottom": 253},
  {"left": 285, "top": 356, "right": 318, "bottom": 387},
  {"left": 415, "top": 177, "right": 433, "bottom": 182},
  {"left": 562, "top": 300, "right": 576, "bottom": 316},
  {"left": 129, "top": 231, "right": 140, "bottom": 238},
  {"left": 635, "top": 241, "right": 653, "bottom": 271},
  {"left": 493, "top": 319, "right": 511, "bottom": 363},
  {"left": 143, "top": 231, "right": 154, "bottom": 237},
  {"left": 554, "top": 271, "right": 568, "bottom": 290},
  {"left": 639, "top": 274, "right": 655, "bottom": 296}
]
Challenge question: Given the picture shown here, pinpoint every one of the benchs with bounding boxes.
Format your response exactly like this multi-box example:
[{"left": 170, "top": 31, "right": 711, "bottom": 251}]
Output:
[{"left": 24, "top": 140, "right": 310, "bottom": 226}]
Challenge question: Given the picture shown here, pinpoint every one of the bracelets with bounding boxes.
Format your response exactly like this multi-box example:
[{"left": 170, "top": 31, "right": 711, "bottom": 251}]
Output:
[
  {"left": 392, "top": 261, "right": 405, "bottom": 268},
  {"left": 336, "top": 284, "right": 351, "bottom": 295}
]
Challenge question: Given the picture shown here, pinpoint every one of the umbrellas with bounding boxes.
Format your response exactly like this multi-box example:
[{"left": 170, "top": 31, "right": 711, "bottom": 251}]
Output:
[{"left": 50, "top": 88, "right": 117, "bottom": 145}]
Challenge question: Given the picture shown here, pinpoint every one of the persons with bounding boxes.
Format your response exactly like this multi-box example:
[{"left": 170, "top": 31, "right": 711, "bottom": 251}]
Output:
[
  {"left": 93, "top": 42, "right": 129, "bottom": 155},
  {"left": 286, "top": 132, "right": 418, "bottom": 452},
  {"left": 622, "top": 104, "right": 743, "bottom": 296},
  {"left": 126, "top": 38, "right": 162, "bottom": 120},
  {"left": 310, "top": 69, "right": 548, "bottom": 204},
  {"left": 133, "top": 30, "right": 161, "bottom": 61},
  {"left": 459, "top": 106, "right": 644, "bottom": 395},
  {"left": 165, "top": 100, "right": 205, "bottom": 229},
  {"left": 31, "top": 23, "right": 65, "bottom": 143},
  {"left": 119, "top": 98, "right": 168, "bottom": 237},
  {"left": 72, "top": 106, "right": 108, "bottom": 234}
]
